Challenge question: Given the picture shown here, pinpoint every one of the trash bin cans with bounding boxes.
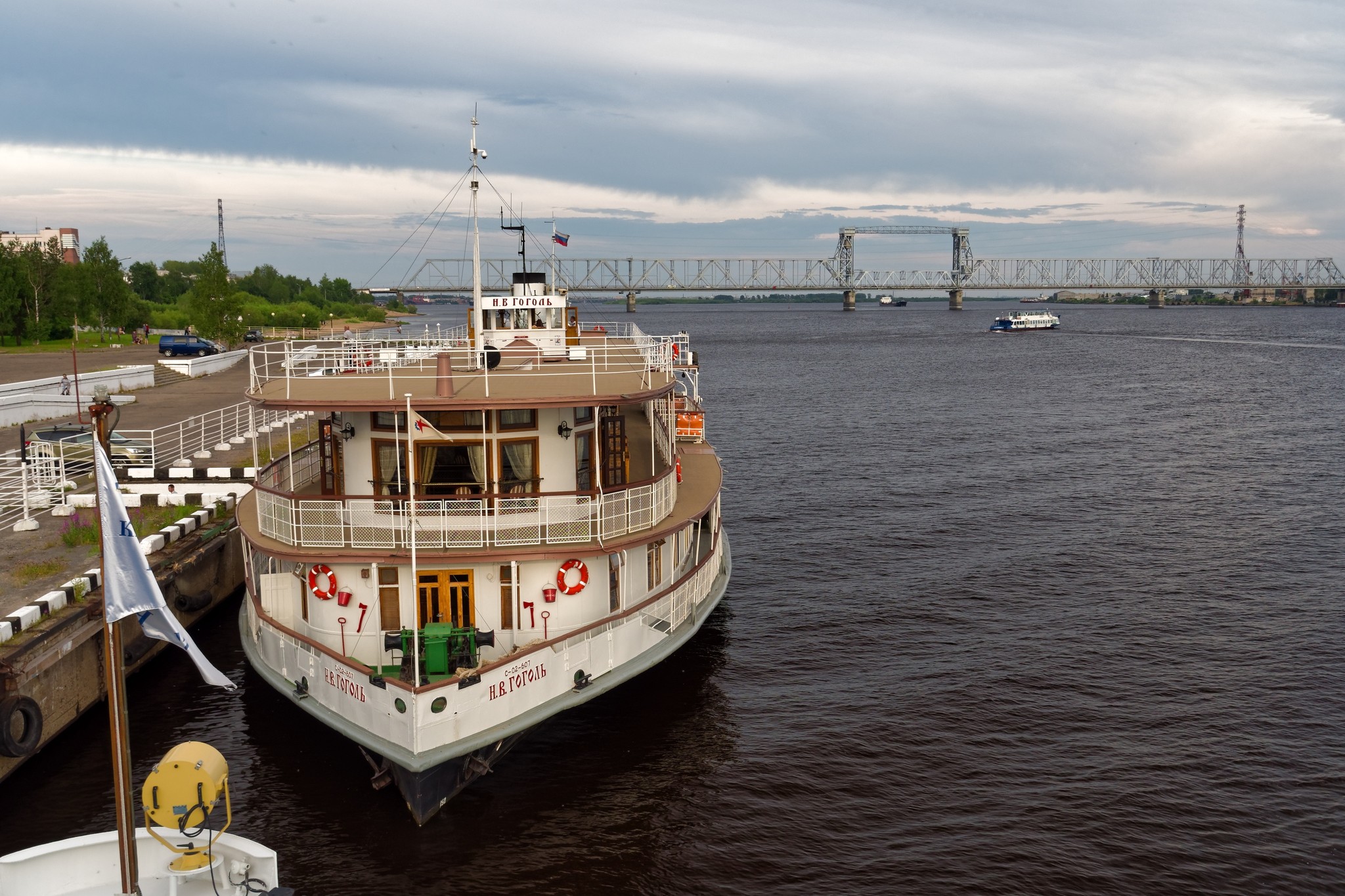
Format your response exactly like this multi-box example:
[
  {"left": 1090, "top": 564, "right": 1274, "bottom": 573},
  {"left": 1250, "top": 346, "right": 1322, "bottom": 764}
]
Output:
[{"left": 677, "top": 412, "right": 702, "bottom": 436}]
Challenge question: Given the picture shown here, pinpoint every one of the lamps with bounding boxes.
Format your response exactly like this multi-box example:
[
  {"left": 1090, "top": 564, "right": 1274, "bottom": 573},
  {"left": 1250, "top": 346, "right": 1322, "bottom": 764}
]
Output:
[
  {"left": 558, "top": 421, "right": 573, "bottom": 440},
  {"left": 339, "top": 422, "right": 355, "bottom": 443}
]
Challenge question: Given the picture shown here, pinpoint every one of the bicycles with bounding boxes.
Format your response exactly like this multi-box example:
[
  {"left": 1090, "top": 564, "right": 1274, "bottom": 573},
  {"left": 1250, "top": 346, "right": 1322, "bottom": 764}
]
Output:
[{"left": 59, "top": 386, "right": 70, "bottom": 395}]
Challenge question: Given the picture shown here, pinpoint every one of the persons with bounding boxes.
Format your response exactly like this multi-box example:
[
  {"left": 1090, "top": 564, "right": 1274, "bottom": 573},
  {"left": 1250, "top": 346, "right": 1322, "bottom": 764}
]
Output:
[
  {"left": 143, "top": 324, "right": 150, "bottom": 333},
  {"left": 144, "top": 331, "right": 149, "bottom": 345},
  {"left": 138, "top": 333, "right": 143, "bottom": 343},
  {"left": 397, "top": 325, "right": 402, "bottom": 334},
  {"left": 561, "top": 315, "right": 570, "bottom": 355},
  {"left": 185, "top": 325, "right": 191, "bottom": 335},
  {"left": 116, "top": 325, "right": 126, "bottom": 335},
  {"left": 58, "top": 374, "right": 72, "bottom": 395},
  {"left": 570, "top": 315, "right": 581, "bottom": 346},
  {"left": 165, "top": 483, "right": 177, "bottom": 494},
  {"left": 132, "top": 330, "right": 140, "bottom": 345},
  {"left": 343, "top": 325, "right": 353, "bottom": 340}
]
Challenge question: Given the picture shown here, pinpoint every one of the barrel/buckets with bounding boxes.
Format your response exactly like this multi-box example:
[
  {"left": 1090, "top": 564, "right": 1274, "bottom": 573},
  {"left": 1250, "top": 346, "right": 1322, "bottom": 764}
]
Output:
[
  {"left": 542, "top": 584, "right": 557, "bottom": 602},
  {"left": 338, "top": 586, "right": 352, "bottom": 607}
]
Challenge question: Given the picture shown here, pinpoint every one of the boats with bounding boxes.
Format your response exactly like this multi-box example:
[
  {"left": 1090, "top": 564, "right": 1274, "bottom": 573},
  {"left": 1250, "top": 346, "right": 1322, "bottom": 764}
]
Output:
[
  {"left": 403, "top": 293, "right": 433, "bottom": 305},
  {"left": 879, "top": 296, "right": 908, "bottom": 307},
  {"left": 1019, "top": 296, "right": 1040, "bottom": 303},
  {"left": 232, "top": 96, "right": 735, "bottom": 832},
  {"left": 0, "top": 338, "right": 297, "bottom": 896},
  {"left": 988, "top": 308, "right": 1062, "bottom": 333}
]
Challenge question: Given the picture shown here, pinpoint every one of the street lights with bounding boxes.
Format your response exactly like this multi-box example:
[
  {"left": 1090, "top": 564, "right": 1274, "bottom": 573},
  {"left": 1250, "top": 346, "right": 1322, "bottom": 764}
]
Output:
[
  {"left": 329, "top": 313, "right": 333, "bottom": 328},
  {"left": 302, "top": 314, "right": 306, "bottom": 327},
  {"left": 271, "top": 313, "right": 275, "bottom": 327},
  {"left": 108, "top": 256, "right": 132, "bottom": 340}
]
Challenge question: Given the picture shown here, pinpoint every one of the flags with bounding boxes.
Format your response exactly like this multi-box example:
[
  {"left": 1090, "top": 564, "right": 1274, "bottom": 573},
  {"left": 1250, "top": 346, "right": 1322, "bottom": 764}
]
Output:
[
  {"left": 555, "top": 230, "right": 571, "bottom": 247},
  {"left": 91, "top": 421, "right": 238, "bottom": 692},
  {"left": 410, "top": 408, "right": 454, "bottom": 444}
]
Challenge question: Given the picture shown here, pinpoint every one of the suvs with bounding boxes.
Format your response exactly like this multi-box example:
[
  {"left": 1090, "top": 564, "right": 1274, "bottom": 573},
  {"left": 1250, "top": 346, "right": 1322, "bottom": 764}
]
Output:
[
  {"left": 158, "top": 334, "right": 215, "bottom": 357},
  {"left": 25, "top": 422, "right": 159, "bottom": 475}
]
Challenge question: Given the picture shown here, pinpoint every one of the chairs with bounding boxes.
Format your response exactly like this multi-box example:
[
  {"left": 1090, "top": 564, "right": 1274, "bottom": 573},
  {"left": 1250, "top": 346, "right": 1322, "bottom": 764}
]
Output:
[
  {"left": 455, "top": 486, "right": 475, "bottom": 512},
  {"left": 508, "top": 484, "right": 526, "bottom": 508}
]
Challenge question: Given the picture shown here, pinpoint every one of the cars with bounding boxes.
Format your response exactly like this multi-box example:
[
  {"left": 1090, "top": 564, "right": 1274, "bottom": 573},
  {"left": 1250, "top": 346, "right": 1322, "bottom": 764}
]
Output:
[
  {"left": 183, "top": 338, "right": 227, "bottom": 356},
  {"left": 243, "top": 330, "right": 265, "bottom": 342}
]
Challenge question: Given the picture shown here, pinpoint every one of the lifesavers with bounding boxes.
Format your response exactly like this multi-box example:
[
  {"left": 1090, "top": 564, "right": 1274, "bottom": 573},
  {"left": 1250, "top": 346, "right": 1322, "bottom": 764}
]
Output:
[
  {"left": 557, "top": 559, "right": 589, "bottom": 595},
  {"left": 353, "top": 348, "right": 372, "bottom": 366},
  {"left": 594, "top": 326, "right": 605, "bottom": 331},
  {"left": 0, "top": 694, "right": 44, "bottom": 758},
  {"left": 309, "top": 563, "right": 337, "bottom": 601},
  {"left": 677, "top": 456, "right": 682, "bottom": 485},
  {"left": 659, "top": 341, "right": 678, "bottom": 361}
]
